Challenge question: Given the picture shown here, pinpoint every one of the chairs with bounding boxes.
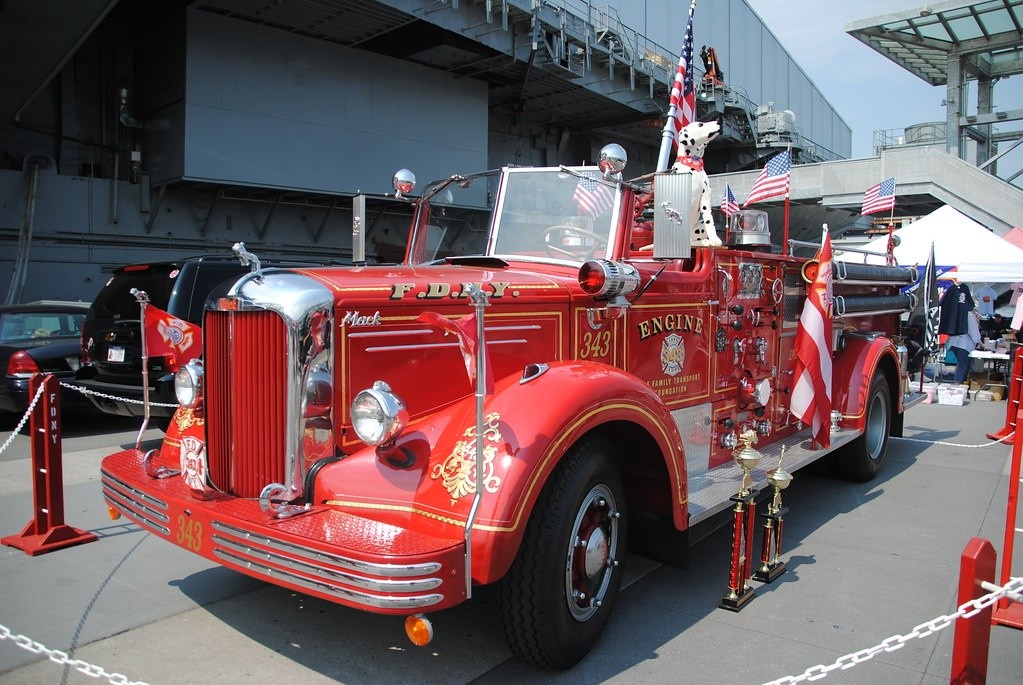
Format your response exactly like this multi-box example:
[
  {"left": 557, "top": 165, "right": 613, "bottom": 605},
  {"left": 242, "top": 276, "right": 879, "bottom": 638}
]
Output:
[{"left": 938, "top": 337, "right": 957, "bottom": 382}]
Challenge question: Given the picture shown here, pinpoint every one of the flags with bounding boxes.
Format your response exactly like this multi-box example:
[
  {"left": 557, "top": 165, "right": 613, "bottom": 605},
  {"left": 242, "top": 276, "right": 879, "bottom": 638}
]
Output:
[
  {"left": 862, "top": 178, "right": 895, "bottom": 216},
  {"left": 790, "top": 232, "right": 832, "bottom": 449},
  {"left": 143, "top": 304, "right": 204, "bottom": 367},
  {"left": 668, "top": 11, "right": 697, "bottom": 151},
  {"left": 574, "top": 172, "right": 615, "bottom": 222},
  {"left": 413, "top": 311, "right": 478, "bottom": 383},
  {"left": 743, "top": 150, "right": 790, "bottom": 207},
  {"left": 721, "top": 185, "right": 740, "bottom": 218},
  {"left": 907, "top": 250, "right": 940, "bottom": 382}
]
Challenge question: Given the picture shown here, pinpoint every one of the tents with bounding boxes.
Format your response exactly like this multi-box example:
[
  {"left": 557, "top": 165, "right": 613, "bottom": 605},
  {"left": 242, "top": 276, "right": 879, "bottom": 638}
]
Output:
[{"left": 832, "top": 204, "right": 1023, "bottom": 282}]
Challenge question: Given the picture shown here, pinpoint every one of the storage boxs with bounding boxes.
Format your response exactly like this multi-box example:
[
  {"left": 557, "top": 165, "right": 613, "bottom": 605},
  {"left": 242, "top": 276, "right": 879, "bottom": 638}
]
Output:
[
  {"left": 909, "top": 381, "right": 939, "bottom": 404},
  {"left": 970, "top": 379, "right": 1005, "bottom": 401},
  {"left": 937, "top": 383, "right": 969, "bottom": 406},
  {"left": 968, "top": 390, "right": 995, "bottom": 401}
]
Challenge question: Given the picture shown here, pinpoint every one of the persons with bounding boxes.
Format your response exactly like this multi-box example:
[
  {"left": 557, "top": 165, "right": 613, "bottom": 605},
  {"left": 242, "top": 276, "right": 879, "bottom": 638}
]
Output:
[
  {"left": 946, "top": 308, "right": 989, "bottom": 384},
  {"left": 991, "top": 315, "right": 1007, "bottom": 341}
]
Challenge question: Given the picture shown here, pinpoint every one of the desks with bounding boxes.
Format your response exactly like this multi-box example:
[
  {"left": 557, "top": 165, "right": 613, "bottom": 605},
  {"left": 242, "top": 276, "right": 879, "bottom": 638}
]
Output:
[{"left": 969, "top": 354, "right": 1010, "bottom": 402}]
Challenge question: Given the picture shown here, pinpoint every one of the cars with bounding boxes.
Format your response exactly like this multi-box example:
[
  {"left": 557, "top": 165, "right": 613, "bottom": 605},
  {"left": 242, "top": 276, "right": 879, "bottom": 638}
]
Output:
[
  {"left": 101, "top": 143, "right": 918, "bottom": 670},
  {"left": 86, "top": 258, "right": 279, "bottom": 436},
  {"left": 0, "top": 302, "right": 90, "bottom": 427}
]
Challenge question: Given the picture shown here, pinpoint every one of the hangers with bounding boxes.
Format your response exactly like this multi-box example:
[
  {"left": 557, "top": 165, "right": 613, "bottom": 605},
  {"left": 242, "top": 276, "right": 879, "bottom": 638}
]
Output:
[{"left": 984, "top": 282, "right": 990, "bottom": 288}]
